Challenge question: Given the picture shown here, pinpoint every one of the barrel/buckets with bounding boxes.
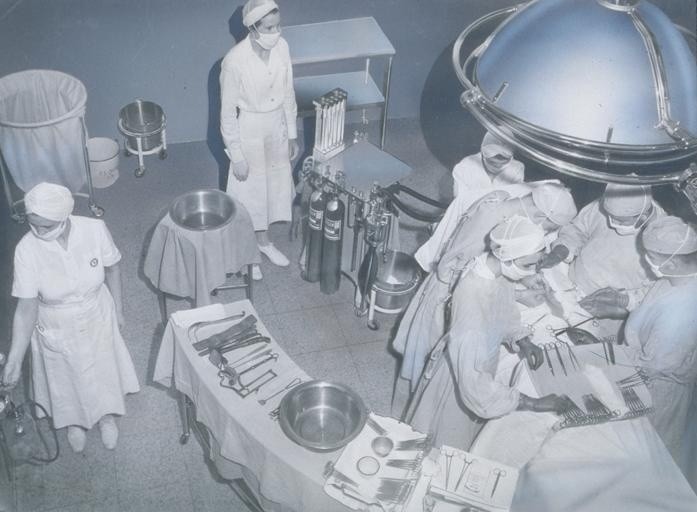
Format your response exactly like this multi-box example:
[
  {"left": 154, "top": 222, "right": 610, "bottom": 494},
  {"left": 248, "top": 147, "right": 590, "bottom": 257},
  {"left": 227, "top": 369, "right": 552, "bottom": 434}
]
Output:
[{"left": 119, "top": 99, "right": 167, "bottom": 152}]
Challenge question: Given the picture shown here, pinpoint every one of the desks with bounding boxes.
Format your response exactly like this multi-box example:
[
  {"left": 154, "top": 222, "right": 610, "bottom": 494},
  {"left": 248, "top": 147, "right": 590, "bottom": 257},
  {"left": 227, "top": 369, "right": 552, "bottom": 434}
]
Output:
[{"left": 165, "top": 299, "right": 519, "bottom": 510}]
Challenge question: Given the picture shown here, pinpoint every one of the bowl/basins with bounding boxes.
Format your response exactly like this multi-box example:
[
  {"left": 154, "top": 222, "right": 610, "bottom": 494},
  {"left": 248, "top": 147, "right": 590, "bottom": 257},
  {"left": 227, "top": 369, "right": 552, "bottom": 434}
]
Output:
[
  {"left": 168, "top": 186, "right": 237, "bottom": 230},
  {"left": 278, "top": 380, "right": 366, "bottom": 453}
]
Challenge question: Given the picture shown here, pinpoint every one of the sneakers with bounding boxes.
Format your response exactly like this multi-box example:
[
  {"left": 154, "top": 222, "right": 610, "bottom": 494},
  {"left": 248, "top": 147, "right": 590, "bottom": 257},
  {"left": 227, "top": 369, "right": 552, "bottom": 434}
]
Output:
[
  {"left": 101, "top": 415, "right": 118, "bottom": 450},
  {"left": 67, "top": 427, "right": 85, "bottom": 453},
  {"left": 247, "top": 264, "right": 263, "bottom": 280},
  {"left": 258, "top": 241, "right": 289, "bottom": 266}
]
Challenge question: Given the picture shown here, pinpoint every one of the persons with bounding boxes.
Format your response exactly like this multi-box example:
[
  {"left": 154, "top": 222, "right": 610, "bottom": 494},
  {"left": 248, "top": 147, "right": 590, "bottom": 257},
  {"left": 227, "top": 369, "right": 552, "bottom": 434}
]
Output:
[
  {"left": 1, "top": 181, "right": 142, "bottom": 454},
  {"left": 537, "top": 172, "right": 696, "bottom": 453},
  {"left": 218, "top": 0, "right": 301, "bottom": 280},
  {"left": 391, "top": 125, "right": 577, "bottom": 450}
]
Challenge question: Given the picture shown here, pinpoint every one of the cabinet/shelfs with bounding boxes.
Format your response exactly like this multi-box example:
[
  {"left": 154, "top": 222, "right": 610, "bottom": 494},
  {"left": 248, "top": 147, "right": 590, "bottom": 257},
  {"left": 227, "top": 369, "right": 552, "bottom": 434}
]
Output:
[{"left": 283, "top": 17, "right": 396, "bottom": 149}]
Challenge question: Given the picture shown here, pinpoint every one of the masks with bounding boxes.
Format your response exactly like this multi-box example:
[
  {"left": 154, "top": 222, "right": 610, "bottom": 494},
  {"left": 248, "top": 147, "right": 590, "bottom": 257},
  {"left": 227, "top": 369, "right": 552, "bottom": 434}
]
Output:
[
  {"left": 248, "top": 24, "right": 281, "bottom": 50},
  {"left": 501, "top": 261, "right": 536, "bottom": 281},
  {"left": 29, "top": 221, "right": 66, "bottom": 242},
  {"left": 645, "top": 254, "right": 671, "bottom": 278},
  {"left": 483, "top": 159, "right": 514, "bottom": 174}
]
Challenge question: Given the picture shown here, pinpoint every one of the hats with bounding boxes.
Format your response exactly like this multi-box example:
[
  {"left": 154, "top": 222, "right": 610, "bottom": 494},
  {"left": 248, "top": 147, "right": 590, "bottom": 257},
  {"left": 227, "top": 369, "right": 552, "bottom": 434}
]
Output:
[
  {"left": 481, "top": 126, "right": 516, "bottom": 158},
  {"left": 24, "top": 182, "right": 74, "bottom": 223},
  {"left": 603, "top": 173, "right": 697, "bottom": 255},
  {"left": 242, "top": 0, "right": 279, "bottom": 27},
  {"left": 489, "top": 185, "right": 576, "bottom": 261}
]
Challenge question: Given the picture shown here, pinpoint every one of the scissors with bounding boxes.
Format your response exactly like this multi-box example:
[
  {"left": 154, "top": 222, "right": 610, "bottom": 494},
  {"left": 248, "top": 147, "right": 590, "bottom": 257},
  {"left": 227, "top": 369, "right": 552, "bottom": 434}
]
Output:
[
  {"left": 545, "top": 324, "right": 567, "bottom": 337},
  {"left": 490, "top": 467, "right": 506, "bottom": 497},
  {"left": 375, "top": 436, "right": 432, "bottom": 504},
  {"left": 556, "top": 366, "right": 654, "bottom": 428},
  {"left": 525, "top": 312, "right": 546, "bottom": 332},
  {"left": 440, "top": 448, "right": 460, "bottom": 490},
  {"left": 600, "top": 334, "right": 617, "bottom": 365},
  {"left": 538, "top": 341, "right": 568, "bottom": 377},
  {"left": 455, "top": 453, "right": 478, "bottom": 491}
]
